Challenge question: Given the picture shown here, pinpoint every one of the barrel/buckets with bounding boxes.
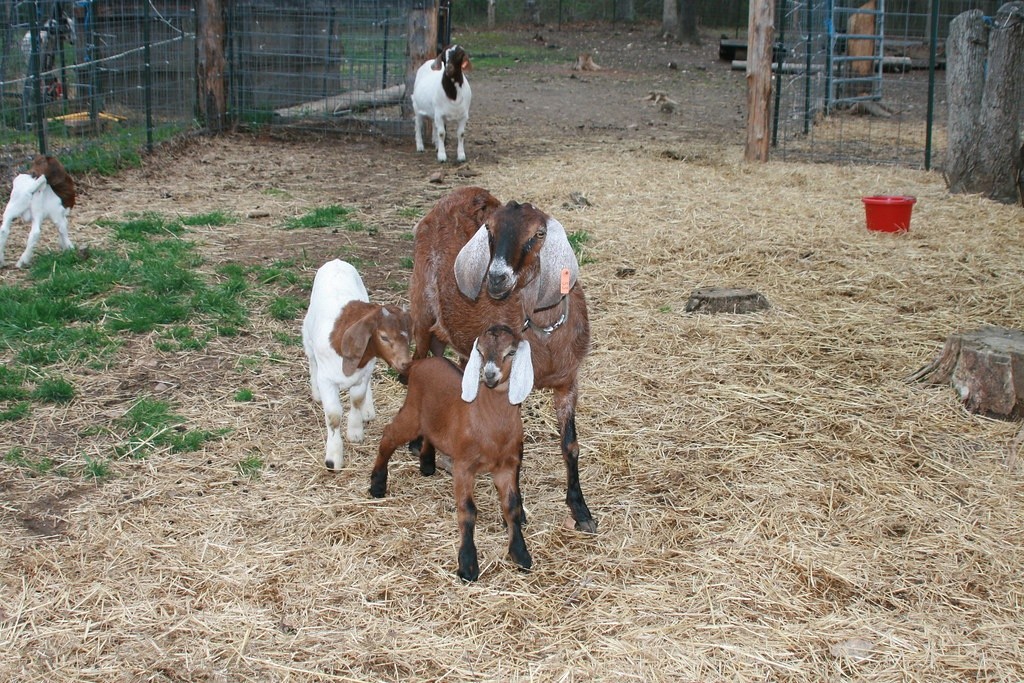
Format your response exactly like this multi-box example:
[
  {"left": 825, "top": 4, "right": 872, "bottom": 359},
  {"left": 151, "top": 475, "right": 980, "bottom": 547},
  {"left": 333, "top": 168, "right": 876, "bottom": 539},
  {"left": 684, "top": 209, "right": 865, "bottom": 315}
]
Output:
[{"left": 862, "top": 197, "right": 917, "bottom": 232}]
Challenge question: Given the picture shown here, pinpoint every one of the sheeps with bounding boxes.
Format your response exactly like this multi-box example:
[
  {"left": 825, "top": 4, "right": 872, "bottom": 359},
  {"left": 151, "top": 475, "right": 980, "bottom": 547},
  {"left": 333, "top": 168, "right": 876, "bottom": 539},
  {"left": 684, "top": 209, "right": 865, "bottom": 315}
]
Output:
[
  {"left": 410, "top": 44, "right": 472, "bottom": 163},
  {"left": 0, "top": 157, "right": 75, "bottom": 271},
  {"left": 20, "top": 10, "right": 78, "bottom": 97},
  {"left": 370, "top": 325, "right": 532, "bottom": 583},
  {"left": 302, "top": 258, "right": 414, "bottom": 474},
  {"left": 399, "top": 187, "right": 596, "bottom": 534}
]
